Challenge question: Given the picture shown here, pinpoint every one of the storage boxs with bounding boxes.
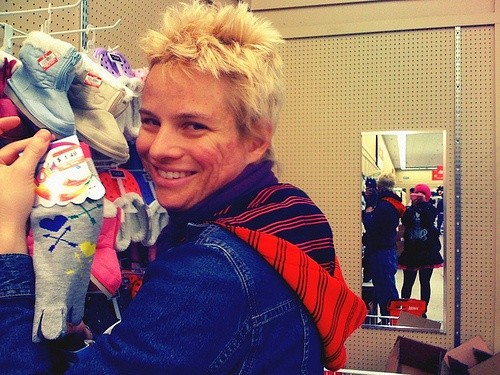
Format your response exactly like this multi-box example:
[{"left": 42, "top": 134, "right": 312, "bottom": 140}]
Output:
[
  {"left": 387, "top": 335, "right": 447, "bottom": 375},
  {"left": 443, "top": 335, "right": 500, "bottom": 375}
]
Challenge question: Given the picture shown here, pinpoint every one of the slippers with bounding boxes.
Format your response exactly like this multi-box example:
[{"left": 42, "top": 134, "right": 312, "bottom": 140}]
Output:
[{"left": 80, "top": 159, "right": 170, "bottom": 299}]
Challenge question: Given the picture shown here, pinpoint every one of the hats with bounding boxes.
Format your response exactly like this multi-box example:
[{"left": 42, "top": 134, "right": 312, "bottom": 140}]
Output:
[{"left": 366, "top": 178, "right": 378, "bottom": 191}]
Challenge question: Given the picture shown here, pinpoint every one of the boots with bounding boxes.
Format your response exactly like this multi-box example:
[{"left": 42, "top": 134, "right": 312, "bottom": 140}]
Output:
[
  {"left": 63, "top": 52, "right": 131, "bottom": 164},
  {"left": 93, "top": 47, "right": 143, "bottom": 142},
  {"left": 3, "top": 31, "right": 83, "bottom": 139}
]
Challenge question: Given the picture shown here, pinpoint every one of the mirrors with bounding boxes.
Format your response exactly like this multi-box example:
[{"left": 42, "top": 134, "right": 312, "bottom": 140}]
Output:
[{"left": 361, "top": 129, "right": 447, "bottom": 335}]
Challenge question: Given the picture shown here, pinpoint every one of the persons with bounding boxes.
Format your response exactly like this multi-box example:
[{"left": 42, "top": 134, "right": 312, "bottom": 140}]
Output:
[
  {"left": 0, "top": 0, "right": 342, "bottom": 375},
  {"left": 362, "top": 174, "right": 440, "bottom": 327}
]
